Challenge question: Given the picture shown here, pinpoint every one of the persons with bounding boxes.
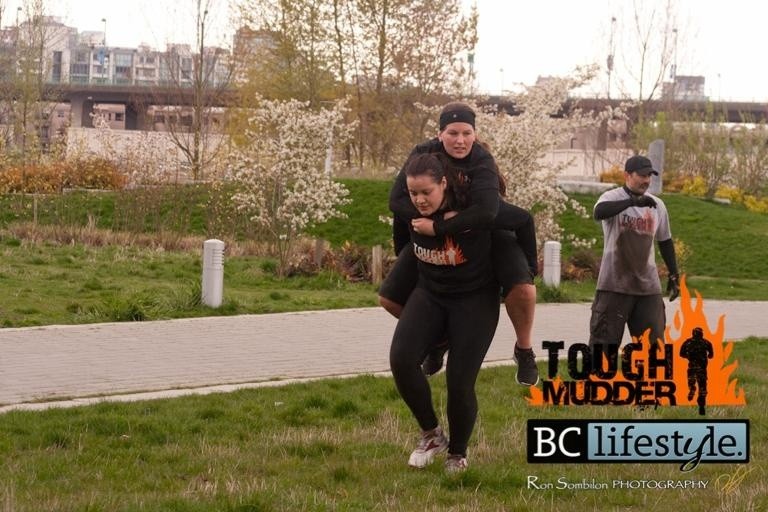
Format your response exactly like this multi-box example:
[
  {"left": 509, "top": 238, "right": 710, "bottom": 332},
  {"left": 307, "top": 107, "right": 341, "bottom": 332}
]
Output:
[
  {"left": 384, "top": 151, "right": 502, "bottom": 473},
  {"left": 583, "top": 152, "right": 682, "bottom": 367},
  {"left": 383, "top": 97, "right": 545, "bottom": 390}
]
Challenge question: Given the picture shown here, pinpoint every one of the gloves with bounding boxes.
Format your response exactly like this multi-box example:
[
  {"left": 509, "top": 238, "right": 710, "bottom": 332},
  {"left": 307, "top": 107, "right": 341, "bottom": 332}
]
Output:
[
  {"left": 666, "top": 273, "right": 680, "bottom": 302},
  {"left": 634, "top": 195, "right": 657, "bottom": 209}
]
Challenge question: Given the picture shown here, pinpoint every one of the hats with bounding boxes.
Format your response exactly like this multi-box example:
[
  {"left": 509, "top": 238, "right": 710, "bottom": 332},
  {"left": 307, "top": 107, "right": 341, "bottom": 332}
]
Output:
[
  {"left": 625, "top": 155, "right": 659, "bottom": 177},
  {"left": 439, "top": 103, "right": 476, "bottom": 131}
]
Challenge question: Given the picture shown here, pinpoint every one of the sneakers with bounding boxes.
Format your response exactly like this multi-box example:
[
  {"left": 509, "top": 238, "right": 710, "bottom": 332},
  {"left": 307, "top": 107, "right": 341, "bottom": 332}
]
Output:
[
  {"left": 407, "top": 425, "right": 450, "bottom": 469},
  {"left": 511, "top": 341, "right": 540, "bottom": 387},
  {"left": 442, "top": 453, "right": 469, "bottom": 479},
  {"left": 421, "top": 340, "right": 450, "bottom": 376}
]
